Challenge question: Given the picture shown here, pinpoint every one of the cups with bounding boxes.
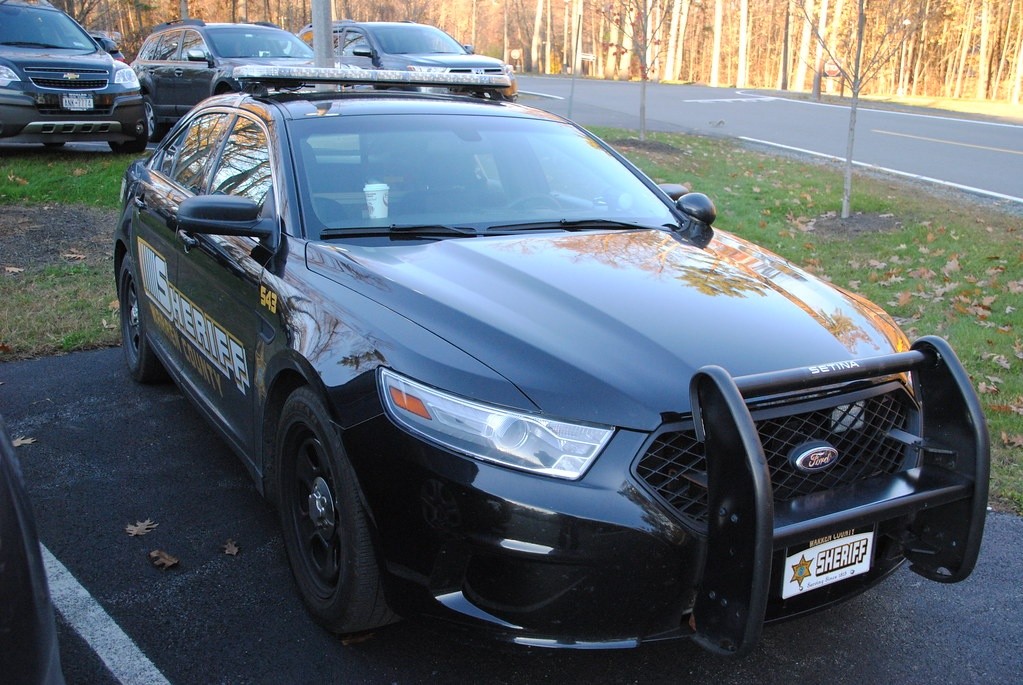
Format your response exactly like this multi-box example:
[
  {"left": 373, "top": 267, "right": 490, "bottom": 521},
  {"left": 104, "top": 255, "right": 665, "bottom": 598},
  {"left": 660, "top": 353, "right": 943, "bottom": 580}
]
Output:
[{"left": 363, "top": 184, "right": 391, "bottom": 217}]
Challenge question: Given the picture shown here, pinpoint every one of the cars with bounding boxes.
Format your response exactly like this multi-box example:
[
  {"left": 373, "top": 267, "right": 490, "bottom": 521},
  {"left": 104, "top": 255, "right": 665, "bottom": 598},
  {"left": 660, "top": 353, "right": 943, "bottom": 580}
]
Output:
[
  {"left": 112, "top": 64, "right": 990, "bottom": 661},
  {"left": 298, "top": 20, "right": 517, "bottom": 102}
]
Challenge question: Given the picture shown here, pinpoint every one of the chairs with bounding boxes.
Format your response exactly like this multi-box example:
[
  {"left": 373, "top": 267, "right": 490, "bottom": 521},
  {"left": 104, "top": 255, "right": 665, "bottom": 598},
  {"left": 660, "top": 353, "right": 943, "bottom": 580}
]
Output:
[{"left": 299, "top": 138, "right": 344, "bottom": 225}]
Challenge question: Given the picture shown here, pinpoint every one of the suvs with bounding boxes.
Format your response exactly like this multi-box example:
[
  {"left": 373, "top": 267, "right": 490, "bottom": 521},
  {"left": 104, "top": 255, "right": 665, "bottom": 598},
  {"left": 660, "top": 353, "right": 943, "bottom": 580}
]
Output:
[
  {"left": 130, "top": 18, "right": 374, "bottom": 144},
  {"left": 0, "top": 0, "right": 149, "bottom": 153}
]
